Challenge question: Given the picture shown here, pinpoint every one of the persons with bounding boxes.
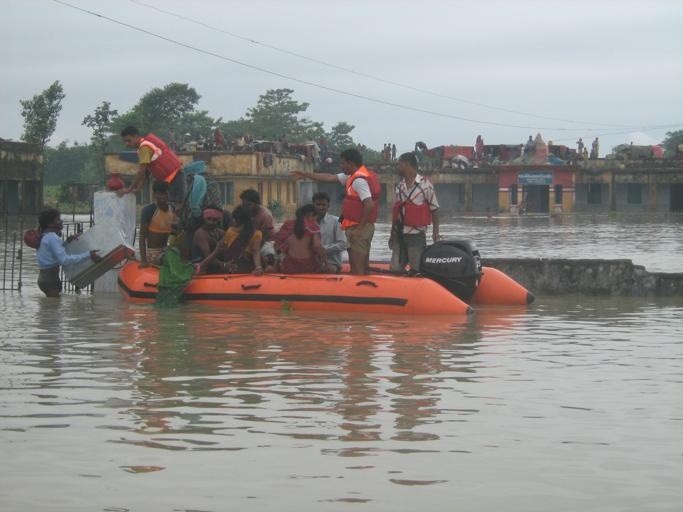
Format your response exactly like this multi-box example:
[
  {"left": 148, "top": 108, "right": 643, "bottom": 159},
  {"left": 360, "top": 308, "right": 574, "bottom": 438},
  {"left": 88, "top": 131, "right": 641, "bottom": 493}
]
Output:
[
  {"left": 288, "top": 150, "right": 381, "bottom": 275},
  {"left": 193, "top": 209, "right": 239, "bottom": 274},
  {"left": 381, "top": 128, "right": 600, "bottom": 168},
  {"left": 117, "top": 127, "right": 183, "bottom": 204},
  {"left": 169, "top": 128, "right": 331, "bottom": 164},
  {"left": 194, "top": 207, "right": 262, "bottom": 276},
  {"left": 139, "top": 183, "right": 178, "bottom": 268},
  {"left": 240, "top": 189, "right": 273, "bottom": 241},
  {"left": 308, "top": 192, "right": 347, "bottom": 273},
  {"left": 36, "top": 209, "right": 103, "bottom": 297},
  {"left": 388, "top": 152, "right": 440, "bottom": 274},
  {"left": 172, "top": 161, "right": 222, "bottom": 257},
  {"left": 274, "top": 205, "right": 321, "bottom": 274}
]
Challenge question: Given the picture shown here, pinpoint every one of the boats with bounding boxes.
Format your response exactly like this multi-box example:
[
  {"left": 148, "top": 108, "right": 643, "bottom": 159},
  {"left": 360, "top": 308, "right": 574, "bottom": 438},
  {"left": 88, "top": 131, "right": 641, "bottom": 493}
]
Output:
[{"left": 118, "top": 262, "right": 535, "bottom": 315}]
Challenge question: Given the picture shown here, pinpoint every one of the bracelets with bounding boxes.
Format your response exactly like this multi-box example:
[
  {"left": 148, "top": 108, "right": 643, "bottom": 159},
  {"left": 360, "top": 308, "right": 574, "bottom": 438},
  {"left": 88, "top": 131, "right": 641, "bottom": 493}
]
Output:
[
  {"left": 204, "top": 257, "right": 210, "bottom": 265},
  {"left": 255, "top": 265, "right": 264, "bottom": 271}
]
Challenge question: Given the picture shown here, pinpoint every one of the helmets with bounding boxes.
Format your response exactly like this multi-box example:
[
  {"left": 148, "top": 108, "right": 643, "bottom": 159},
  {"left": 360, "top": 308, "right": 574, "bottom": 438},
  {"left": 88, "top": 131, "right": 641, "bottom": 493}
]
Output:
[{"left": 24, "top": 229, "right": 40, "bottom": 248}]
